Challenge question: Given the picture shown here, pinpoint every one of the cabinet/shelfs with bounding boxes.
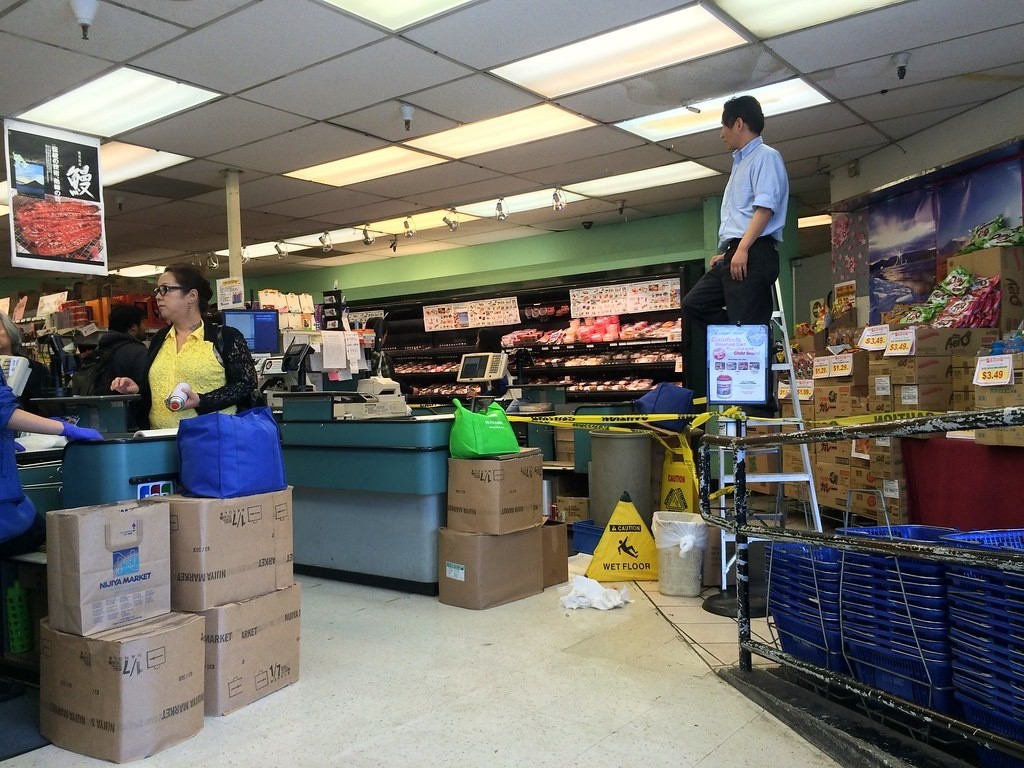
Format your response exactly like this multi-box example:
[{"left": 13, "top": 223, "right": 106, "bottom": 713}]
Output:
[{"left": 382, "top": 336, "right": 681, "bottom": 404}]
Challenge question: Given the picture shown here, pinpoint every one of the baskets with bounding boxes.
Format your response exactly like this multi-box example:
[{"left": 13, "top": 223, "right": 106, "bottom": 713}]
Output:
[{"left": 762, "top": 525, "right": 1024, "bottom": 743}]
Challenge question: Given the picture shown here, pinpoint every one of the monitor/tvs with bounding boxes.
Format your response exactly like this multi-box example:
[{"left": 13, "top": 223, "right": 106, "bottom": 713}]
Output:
[
  {"left": 460, "top": 355, "right": 489, "bottom": 378},
  {"left": 221, "top": 309, "right": 280, "bottom": 355}
]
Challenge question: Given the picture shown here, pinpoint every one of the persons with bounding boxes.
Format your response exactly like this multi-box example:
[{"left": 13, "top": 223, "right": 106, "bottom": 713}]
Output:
[
  {"left": 0, "top": 312, "right": 66, "bottom": 417},
  {"left": 0, "top": 368, "right": 105, "bottom": 704},
  {"left": 365, "top": 317, "right": 414, "bottom": 396},
  {"left": 680, "top": 96, "right": 790, "bottom": 446},
  {"left": 110, "top": 263, "right": 258, "bottom": 430},
  {"left": 49, "top": 302, "right": 150, "bottom": 433},
  {"left": 466, "top": 327, "right": 509, "bottom": 400}
]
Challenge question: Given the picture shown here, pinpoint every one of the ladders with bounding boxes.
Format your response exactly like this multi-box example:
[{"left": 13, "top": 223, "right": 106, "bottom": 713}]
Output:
[{"left": 720, "top": 279, "right": 823, "bottom": 598}]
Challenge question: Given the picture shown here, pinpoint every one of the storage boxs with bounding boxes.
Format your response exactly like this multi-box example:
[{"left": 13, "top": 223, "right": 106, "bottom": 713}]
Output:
[
  {"left": 180, "top": 579, "right": 301, "bottom": 717},
  {"left": 742, "top": 247, "right": 1022, "bottom": 528},
  {"left": 438, "top": 522, "right": 542, "bottom": 609},
  {"left": 571, "top": 518, "right": 606, "bottom": 554},
  {"left": 556, "top": 494, "right": 590, "bottom": 524},
  {"left": 446, "top": 446, "right": 544, "bottom": 535},
  {"left": 144, "top": 483, "right": 294, "bottom": 612},
  {"left": 38, "top": 613, "right": 206, "bottom": 763}
]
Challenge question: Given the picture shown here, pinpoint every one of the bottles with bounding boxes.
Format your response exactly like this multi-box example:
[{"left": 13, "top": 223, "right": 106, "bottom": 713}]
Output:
[{"left": 167, "top": 382, "right": 191, "bottom": 411}]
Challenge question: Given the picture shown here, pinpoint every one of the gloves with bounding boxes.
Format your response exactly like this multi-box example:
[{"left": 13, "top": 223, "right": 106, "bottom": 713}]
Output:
[
  {"left": 60, "top": 420, "right": 103, "bottom": 442},
  {"left": 12, "top": 441, "right": 26, "bottom": 452}
]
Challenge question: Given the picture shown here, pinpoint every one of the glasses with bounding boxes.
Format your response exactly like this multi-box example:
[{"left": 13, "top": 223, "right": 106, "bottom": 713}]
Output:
[{"left": 154, "top": 285, "right": 184, "bottom": 297}]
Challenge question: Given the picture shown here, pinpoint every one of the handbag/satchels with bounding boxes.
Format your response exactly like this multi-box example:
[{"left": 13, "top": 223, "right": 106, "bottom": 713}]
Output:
[
  {"left": 449, "top": 397, "right": 520, "bottom": 458},
  {"left": 634, "top": 382, "right": 694, "bottom": 431},
  {"left": 177, "top": 408, "right": 286, "bottom": 499}
]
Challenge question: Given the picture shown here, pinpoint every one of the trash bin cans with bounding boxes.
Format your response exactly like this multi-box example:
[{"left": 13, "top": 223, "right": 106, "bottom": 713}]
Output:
[{"left": 654, "top": 511, "right": 706, "bottom": 597}]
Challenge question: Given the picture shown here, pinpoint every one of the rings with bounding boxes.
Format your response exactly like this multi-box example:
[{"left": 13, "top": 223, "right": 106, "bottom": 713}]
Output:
[{"left": 738, "top": 268, "right": 742, "bottom": 271}]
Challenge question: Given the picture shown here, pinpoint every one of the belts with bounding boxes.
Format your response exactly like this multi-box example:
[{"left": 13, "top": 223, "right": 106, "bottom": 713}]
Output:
[{"left": 726, "top": 235, "right": 773, "bottom": 251}]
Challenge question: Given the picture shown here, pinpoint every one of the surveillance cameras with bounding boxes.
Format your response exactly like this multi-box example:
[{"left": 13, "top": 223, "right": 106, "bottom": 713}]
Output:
[{"left": 582, "top": 221, "right": 593, "bottom": 230}]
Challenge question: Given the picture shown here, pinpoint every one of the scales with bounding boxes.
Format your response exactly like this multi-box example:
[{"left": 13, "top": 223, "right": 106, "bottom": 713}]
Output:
[{"left": 457, "top": 352, "right": 508, "bottom": 412}]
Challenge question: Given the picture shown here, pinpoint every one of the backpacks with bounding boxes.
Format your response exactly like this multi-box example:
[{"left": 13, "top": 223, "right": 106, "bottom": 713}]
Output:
[{"left": 72, "top": 340, "right": 147, "bottom": 396}]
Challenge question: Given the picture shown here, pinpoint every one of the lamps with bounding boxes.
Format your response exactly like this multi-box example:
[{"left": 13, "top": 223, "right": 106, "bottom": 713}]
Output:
[{"left": 206, "top": 184, "right": 566, "bottom": 270}]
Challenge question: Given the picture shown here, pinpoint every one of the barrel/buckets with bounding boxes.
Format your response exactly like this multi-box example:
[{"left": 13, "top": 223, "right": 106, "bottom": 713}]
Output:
[{"left": 588, "top": 428, "right": 655, "bottom": 527}]
